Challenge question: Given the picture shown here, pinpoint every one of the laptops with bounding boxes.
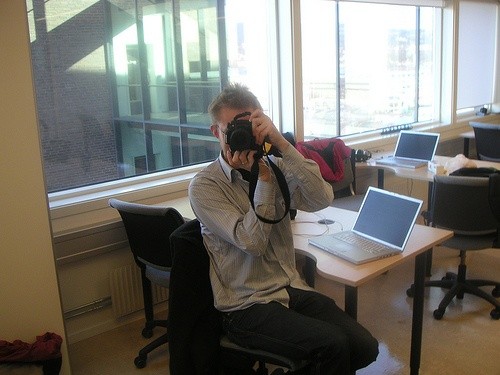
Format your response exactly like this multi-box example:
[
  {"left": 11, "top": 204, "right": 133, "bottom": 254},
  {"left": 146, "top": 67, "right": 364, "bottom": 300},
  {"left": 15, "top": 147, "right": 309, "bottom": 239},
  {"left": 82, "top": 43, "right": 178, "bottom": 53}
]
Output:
[
  {"left": 308, "top": 186, "right": 424, "bottom": 265},
  {"left": 376, "top": 130, "right": 441, "bottom": 169}
]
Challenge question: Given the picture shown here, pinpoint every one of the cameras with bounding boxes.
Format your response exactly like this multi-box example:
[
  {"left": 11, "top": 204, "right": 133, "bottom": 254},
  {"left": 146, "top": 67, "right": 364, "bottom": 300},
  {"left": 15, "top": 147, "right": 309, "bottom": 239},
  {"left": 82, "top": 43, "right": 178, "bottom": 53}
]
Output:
[{"left": 224, "top": 120, "right": 264, "bottom": 160}]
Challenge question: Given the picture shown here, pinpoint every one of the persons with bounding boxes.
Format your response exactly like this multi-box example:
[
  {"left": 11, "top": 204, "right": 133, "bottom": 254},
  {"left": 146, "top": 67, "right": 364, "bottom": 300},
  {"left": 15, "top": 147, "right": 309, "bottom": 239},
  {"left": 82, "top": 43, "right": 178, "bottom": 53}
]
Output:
[{"left": 188, "top": 83, "right": 381, "bottom": 375}]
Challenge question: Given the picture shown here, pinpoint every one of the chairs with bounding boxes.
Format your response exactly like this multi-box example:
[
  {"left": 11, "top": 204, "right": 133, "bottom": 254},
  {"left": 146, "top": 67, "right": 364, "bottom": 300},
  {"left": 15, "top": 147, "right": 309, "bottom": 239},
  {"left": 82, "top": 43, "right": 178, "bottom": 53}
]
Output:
[
  {"left": 168, "top": 219, "right": 297, "bottom": 375},
  {"left": 106, "top": 198, "right": 186, "bottom": 369},
  {"left": 407, "top": 176, "right": 500, "bottom": 321},
  {"left": 468, "top": 122, "right": 500, "bottom": 162}
]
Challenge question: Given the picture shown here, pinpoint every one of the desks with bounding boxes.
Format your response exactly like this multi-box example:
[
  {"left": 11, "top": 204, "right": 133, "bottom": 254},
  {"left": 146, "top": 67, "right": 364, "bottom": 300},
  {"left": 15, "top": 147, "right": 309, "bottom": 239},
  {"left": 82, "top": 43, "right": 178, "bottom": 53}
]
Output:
[
  {"left": 459, "top": 130, "right": 475, "bottom": 158},
  {"left": 152, "top": 196, "right": 453, "bottom": 375},
  {"left": 352, "top": 151, "right": 500, "bottom": 227}
]
